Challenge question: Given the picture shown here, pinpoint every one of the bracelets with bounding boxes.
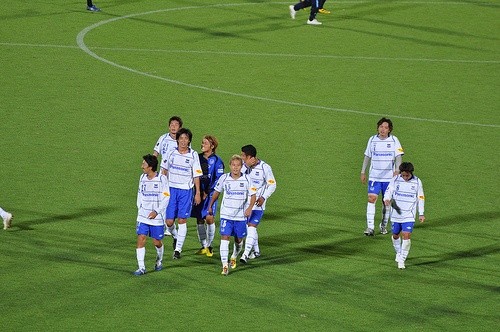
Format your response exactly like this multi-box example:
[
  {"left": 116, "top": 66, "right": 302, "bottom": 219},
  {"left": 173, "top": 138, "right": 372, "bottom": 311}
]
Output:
[{"left": 261, "top": 196, "right": 265, "bottom": 200}]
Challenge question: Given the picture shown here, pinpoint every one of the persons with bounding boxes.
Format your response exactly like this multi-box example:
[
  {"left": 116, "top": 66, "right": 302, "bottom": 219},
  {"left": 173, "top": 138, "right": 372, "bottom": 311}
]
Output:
[
  {"left": 360, "top": 116, "right": 405, "bottom": 236},
  {"left": 190, "top": 135, "right": 226, "bottom": 257},
  {"left": 86, "top": 0, "right": 99, "bottom": 12},
  {"left": 239, "top": 144, "right": 277, "bottom": 263},
  {"left": 208, "top": 154, "right": 257, "bottom": 276},
  {"left": 300, "top": 0, "right": 331, "bottom": 14},
  {"left": 160, "top": 127, "right": 203, "bottom": 258},
  {"left": 0, "top": 206, "right": 14, "bottom": 230},
  {"left": 383, "top": 160, "right": 425, "bottom": 270},
  {"left": 289, "top": 0, "right": 326, "bottom": 25},
  {"left": 132, "top": 153, "right": 170, "bottom": 274},
  {"left": 152, "top": 116, "right": 191, "bottom": 236}
]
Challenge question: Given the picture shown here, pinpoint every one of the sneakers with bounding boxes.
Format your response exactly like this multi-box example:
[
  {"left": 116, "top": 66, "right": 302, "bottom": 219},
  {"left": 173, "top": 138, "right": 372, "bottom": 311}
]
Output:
[
  {"left": 364, "top": 228, "right": 374, "bottom": 236},
  {"left": 3, "top": 213, "right": 14, "bottom": 230},
  {"left": 307, "top": 19, "right": 322, "bottom": 25},
  {"left": 379, "top": 223, "right": 388, "bottom": 235},
  {"left": 155, "top": 257, "right": 162, "bottom": 271},
  {"left": 395, "top": 253, "right": 400, "bottom": 262},
  {"left": 247, "top": 250, "right": 261, "bottom": 260},
  {"left": 318, "top": 8, "right": 330, "bottom": 14},
  {"left": 195, "top": 247, "right": 205, "bottom": 255},
  {"left": 87, "top": 5, "right": 101, "bottom": 12},
  {"left": 221, "top": 266, "right": 228, "bottom": 275},
  {"left": 164, "top": 230, "right": 172, "bottom": 236},
  {"left": 300, "top": 0, "right": 307, "bottom": 11},
  {"left": 398, "top": 260, "right": 406, "bottom": 269},
  {"left": 173, "top": 238, "right": 177, "bottom": 250},
  {"left": 239, "top": 254, "right": 248, "bottom": 264},
  {"left": 229, "top": 257, "right": 236, "bottom": 269},
  {"left": 173, "top": 251, "right": 180, "bottom": 260},
  {"left": 289, "top": 5, "right": 296, "bottom": 20},
  {"left": 206, "top": 246, "right": 214, "bottom": 257},
  {"left": 135, "top": 268, "right": 147, "bottom": 275}
]
